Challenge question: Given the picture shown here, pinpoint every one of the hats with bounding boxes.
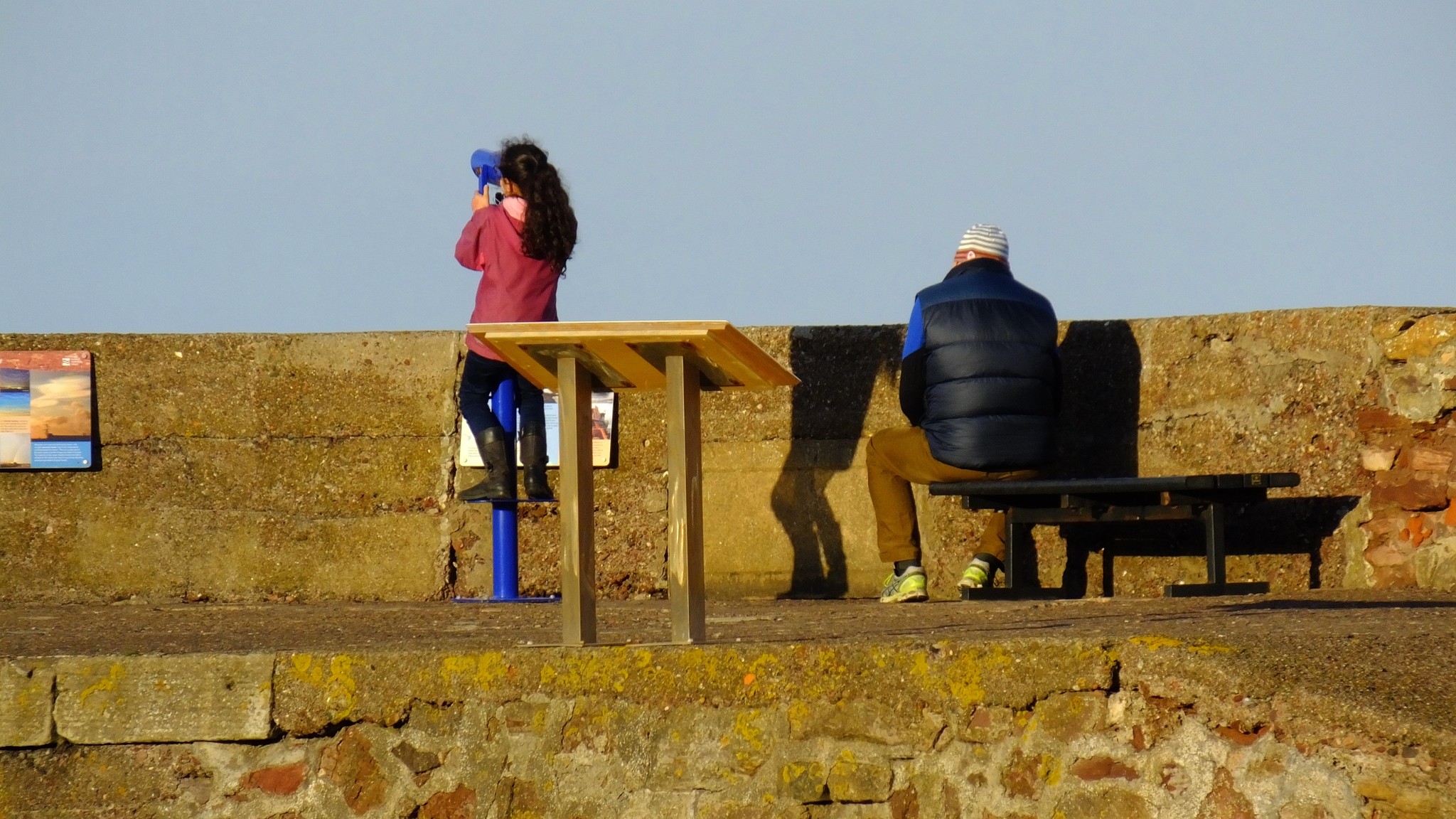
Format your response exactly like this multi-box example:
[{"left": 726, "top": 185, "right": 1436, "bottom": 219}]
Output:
[{"left": 953, "top": 224, "right": 1010, "bottom": 266}]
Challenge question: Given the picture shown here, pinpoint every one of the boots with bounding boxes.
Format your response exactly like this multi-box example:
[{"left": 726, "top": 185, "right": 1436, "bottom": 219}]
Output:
[
  {"left": 520, "top": 425, "right": 554, "bottom": 500},
  {"left": 456, "top": 427, "right": 516, "bottom": 501}
]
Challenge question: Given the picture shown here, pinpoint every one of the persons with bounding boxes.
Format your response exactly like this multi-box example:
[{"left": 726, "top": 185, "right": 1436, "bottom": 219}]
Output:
[
  {"left": 454, "top": 137, "right": 578, "bottom": 501},
  {"left": 866, "top": 224, "right": 1057, "bottom": 603}
]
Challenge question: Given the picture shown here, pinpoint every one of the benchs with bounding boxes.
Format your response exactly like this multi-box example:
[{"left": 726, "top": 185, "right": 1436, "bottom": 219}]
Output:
[{"left": 928, "top": 473, "right": 1302, "bottom": 592}]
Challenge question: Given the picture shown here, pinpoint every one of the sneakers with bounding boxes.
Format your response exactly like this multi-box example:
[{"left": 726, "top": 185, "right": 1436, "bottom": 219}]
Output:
[
  {"left": 957, "top": 557, "right": 999, "bottom": 595},
  {"left": 879, "top": 564, "right": 929, "bottom": 602}
]
